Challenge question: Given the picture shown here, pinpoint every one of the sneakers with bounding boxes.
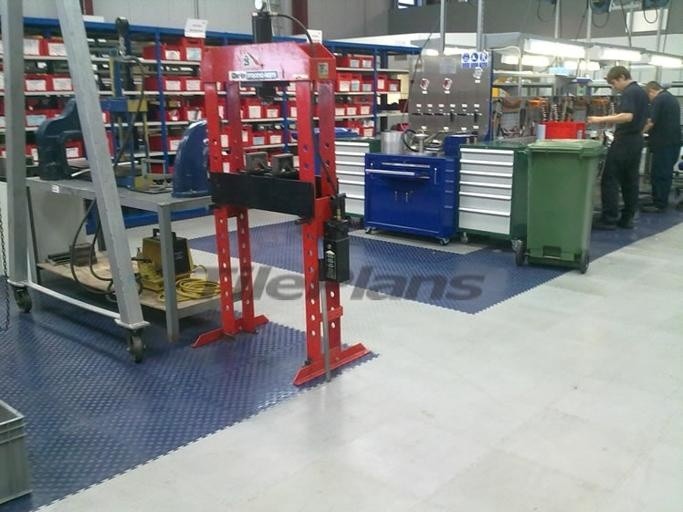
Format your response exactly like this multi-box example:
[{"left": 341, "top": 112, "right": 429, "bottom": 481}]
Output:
[
  {"left": 638, "top": 200, "right": 663, "bottom": 212},
  {"left": 593, "top": 219, "right": 616, "bottom": 229},
  {"left": 620, "top": 215, "right": 632, "bottom": 227}
]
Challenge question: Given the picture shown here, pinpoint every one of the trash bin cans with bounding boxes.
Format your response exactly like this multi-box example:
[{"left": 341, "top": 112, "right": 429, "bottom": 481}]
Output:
[
  {"left": 516, "top": 138, "right": 608, "bottom": 274},
  {"left": 0, "top": 399, "right": 33, "bottom": 512}
]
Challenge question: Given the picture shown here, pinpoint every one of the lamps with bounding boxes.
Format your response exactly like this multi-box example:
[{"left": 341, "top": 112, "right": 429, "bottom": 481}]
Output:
[{"left": 500, "top": 0, "right": 683, "bottom": 71}]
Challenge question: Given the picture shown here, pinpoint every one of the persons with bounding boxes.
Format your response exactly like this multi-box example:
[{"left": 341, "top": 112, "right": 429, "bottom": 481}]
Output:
[
  {"left": 640, "top": 79, "right": 681, "bottom": 214},
  {"left": 586, "top": 65, "right": 650, "bottom": 232}
]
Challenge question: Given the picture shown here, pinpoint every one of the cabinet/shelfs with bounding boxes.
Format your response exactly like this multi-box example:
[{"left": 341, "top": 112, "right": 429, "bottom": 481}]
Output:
[{"left": 0, "top": 16, "right": 424, "bottom": 347}]
